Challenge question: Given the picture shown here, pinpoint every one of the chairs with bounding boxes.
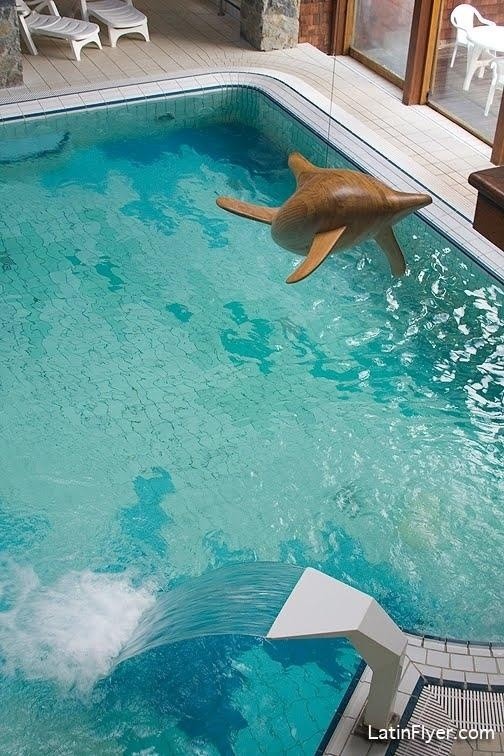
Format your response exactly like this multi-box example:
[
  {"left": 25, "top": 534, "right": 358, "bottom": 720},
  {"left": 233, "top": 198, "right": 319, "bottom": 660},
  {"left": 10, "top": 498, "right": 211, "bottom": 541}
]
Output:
[
  {"left": 448, "top": 2, "right": 504, "bottom": 117},
  {"left": 15, "top": 0, "right": 150, "bottom": 62}
]
